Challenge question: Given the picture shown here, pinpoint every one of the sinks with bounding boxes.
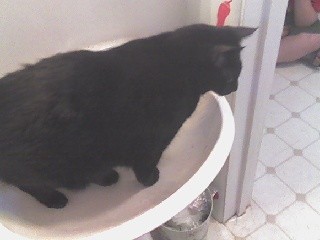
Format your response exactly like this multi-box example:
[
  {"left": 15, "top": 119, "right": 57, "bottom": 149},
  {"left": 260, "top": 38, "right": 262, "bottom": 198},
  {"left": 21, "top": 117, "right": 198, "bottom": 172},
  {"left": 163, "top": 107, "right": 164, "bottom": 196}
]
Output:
[{"left": 0, "top": 38, "right": 235, "bottom": 240}]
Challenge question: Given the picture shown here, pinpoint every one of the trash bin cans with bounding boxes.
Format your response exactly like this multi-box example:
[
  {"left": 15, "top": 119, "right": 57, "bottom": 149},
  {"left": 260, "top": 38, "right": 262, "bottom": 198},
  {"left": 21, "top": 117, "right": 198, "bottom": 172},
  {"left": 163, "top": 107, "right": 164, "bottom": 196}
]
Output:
[{"left": 150, "top": 187, "right": 214, "bottom": 240}]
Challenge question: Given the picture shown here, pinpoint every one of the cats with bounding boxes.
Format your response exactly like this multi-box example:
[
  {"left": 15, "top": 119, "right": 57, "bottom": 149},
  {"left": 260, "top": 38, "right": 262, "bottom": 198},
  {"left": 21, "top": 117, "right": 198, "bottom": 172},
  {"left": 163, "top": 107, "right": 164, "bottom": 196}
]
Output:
[{"left": 0, "top": 23, "right": 260, "bottom": 209}]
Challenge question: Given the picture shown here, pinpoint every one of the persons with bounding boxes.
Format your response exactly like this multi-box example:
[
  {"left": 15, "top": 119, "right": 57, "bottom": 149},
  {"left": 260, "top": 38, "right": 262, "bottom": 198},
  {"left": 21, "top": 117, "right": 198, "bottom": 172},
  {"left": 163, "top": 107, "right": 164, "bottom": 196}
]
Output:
[{"left": 275, "top": 0, "right": 320, "bottom": 68}]
